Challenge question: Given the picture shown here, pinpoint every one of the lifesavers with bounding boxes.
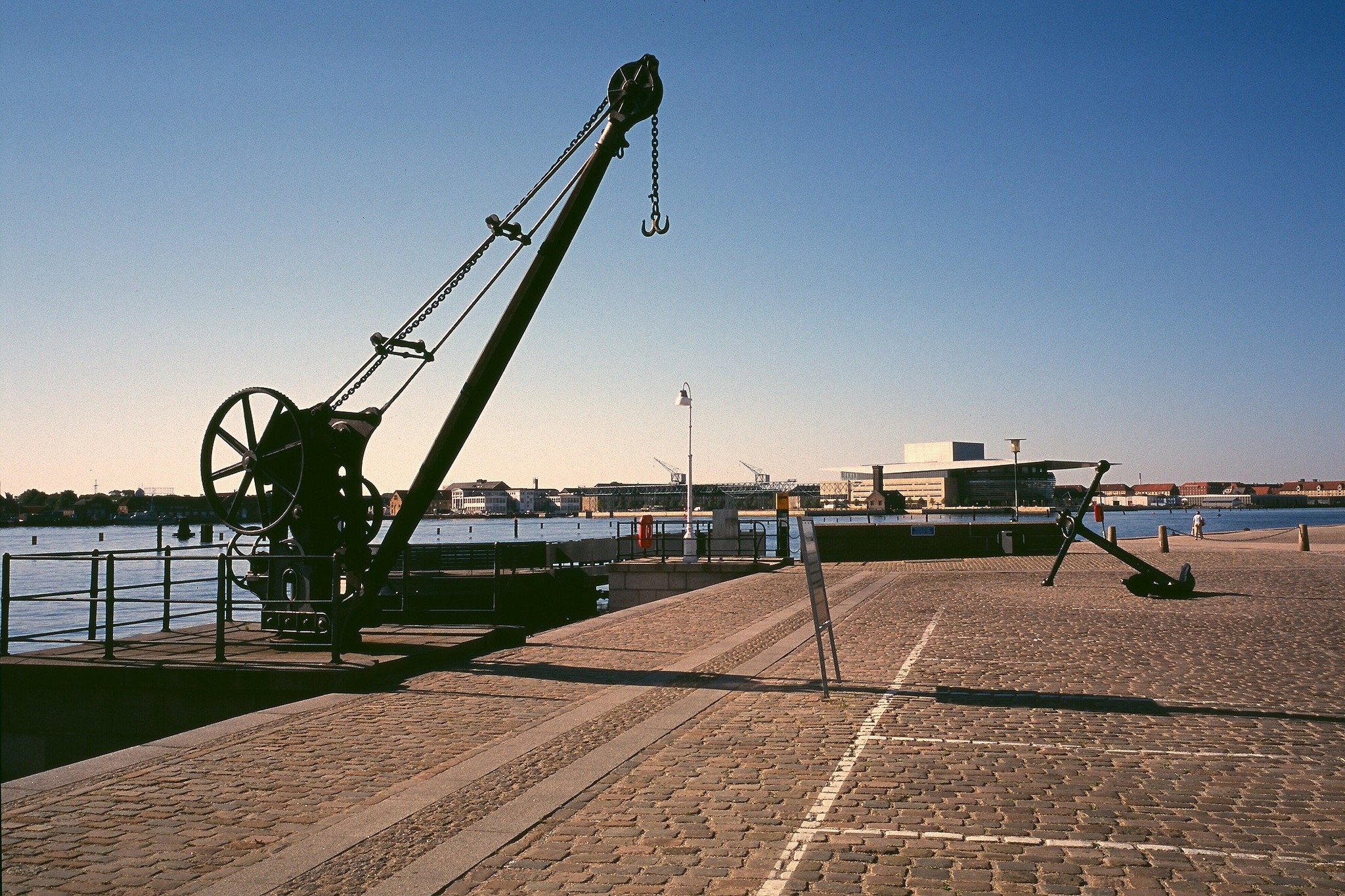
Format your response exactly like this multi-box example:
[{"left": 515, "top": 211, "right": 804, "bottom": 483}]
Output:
[{"left": 638, "top": 515, "right": 653, "bottom": 548}]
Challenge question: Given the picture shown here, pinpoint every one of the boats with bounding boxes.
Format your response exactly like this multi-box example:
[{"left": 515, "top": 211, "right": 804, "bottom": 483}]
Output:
[{"left": 114, "top": 499, "right": 177, "bottom": 525}]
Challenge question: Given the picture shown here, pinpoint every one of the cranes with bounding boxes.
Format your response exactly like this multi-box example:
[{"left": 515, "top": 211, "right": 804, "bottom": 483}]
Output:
[
  {"left": 653, "top": 458, "right": 686, "bottom": 484},
  {"left": 739, "top": 460, "right": 770, "bottom": 485}
]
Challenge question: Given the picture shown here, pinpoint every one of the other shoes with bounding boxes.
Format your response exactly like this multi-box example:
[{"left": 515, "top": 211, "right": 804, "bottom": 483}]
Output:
[
  {"left": 1195, "top": 537, "right": 1197, "bottom": 540},
  {"left": 1201, "top": 537, "right": 1203, "bottom": 540}
]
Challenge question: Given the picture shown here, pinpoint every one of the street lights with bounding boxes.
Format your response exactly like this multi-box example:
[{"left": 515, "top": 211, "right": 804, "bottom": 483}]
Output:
[
  {"left": 1005, "top": 439, "right": 1026, "bottom": 522},
  {"left": 676, "top": 382, "right": 698, "bottom": 563},
  {"left": 17, "top": 502, "right": 21, "bottom": 514}
]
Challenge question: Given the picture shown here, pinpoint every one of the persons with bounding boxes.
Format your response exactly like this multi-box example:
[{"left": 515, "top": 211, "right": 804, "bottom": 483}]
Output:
[{"left": 1192, "top": 511, "right": 1204, "bottom": 540}]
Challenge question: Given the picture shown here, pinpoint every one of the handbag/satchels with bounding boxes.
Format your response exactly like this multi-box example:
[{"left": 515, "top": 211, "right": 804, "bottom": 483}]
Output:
[
  {"left": 1191, "top": 526, "right": 1194, "bottom": 535},
  {"left": 1200, "top": 515, "right": 1204, "bottom": 526}
]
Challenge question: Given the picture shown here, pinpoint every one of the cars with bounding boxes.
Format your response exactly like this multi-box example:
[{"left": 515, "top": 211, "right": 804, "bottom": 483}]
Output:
[{"left": 1097, "top": 503, "right": 1104, "bottom": 505}]
